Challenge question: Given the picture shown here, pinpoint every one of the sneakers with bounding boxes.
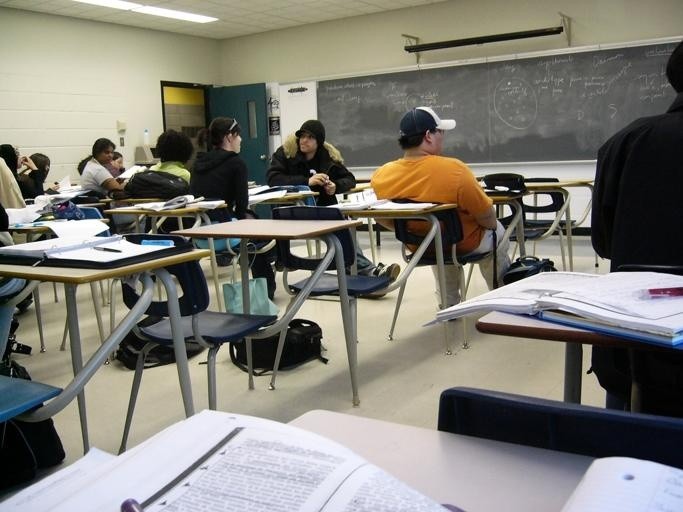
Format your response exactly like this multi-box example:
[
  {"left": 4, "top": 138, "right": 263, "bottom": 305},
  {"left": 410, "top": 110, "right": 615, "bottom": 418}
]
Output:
[{"left": 373, "top": 261, "right": 400, "bottom": 282}]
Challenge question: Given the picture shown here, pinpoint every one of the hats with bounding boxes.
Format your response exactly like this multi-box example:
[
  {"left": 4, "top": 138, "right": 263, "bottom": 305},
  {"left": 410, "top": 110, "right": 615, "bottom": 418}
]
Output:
[
  {"left": 294, "top": 120, "right": 325, "bottom": 144},
  {"left": 400, "top": 106, "right": 456, "bottom": 136}
]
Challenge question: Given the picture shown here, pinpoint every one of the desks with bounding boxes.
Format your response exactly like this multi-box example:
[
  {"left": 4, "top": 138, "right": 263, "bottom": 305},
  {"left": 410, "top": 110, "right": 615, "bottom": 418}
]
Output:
[
  {"left": 475, "top": 311, "right": 681, "bottom": 404},
  {"left": 286, "top": 408, "right": 596, "bottom": 512}
]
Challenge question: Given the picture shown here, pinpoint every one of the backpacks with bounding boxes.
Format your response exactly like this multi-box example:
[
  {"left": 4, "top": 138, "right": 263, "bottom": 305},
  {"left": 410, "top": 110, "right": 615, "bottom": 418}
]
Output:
[
  {"left": 229, "top": 319, "right": 328, "bottom": 377},
  {"left": 114, "top": 312, "right": 213, "bottom": 371},
  {"left": 106, "top": 169, "right": 189, "bottom": 199}
]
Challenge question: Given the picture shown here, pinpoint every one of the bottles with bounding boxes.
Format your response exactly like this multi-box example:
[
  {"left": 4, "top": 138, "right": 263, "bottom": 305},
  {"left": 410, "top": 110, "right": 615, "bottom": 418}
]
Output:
[{"left": 144, "top": 129, "right": 150, "bottom": 145}]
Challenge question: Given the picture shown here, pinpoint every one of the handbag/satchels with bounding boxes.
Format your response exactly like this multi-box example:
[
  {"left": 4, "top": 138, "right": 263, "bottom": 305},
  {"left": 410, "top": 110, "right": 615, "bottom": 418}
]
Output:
[
  {"left": 504, "top": 255, "right": 558, "bottom": 286},
  {"left": 223, "top": 243, "right": 281, "bottom": 322},
  {"left": 0, "top": 347, "right": 67, "bottom": 496}
]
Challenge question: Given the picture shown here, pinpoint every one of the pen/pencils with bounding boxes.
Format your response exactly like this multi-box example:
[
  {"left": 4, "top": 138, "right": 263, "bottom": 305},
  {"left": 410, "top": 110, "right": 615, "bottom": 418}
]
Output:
[
  {"left": 310, "top": 169, "right": 330, "bottom": 184},
  {"left": 641, "top": 287, "right": 683, "bottom": 297},
  {"left": 92, "top": 246, "right": 121, "bottom": 252}
]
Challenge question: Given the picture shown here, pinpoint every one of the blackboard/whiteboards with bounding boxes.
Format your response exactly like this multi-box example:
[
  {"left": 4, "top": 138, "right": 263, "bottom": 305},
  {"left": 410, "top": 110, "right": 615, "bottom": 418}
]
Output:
[{"left": 315, "top": 36, "right": 683, "bottom": 171}]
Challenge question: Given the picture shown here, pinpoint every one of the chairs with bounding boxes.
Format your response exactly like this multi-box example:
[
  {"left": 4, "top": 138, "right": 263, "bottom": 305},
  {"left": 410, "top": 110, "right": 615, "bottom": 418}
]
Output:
[
  {"left": 0, "top": 172, "right": 594, "bottom": 457},
  {"left": 436, "top": 387, "right": 683, "bottom": 471},
  {"left": 611, "top": 263, "right": 683, "bottom": 414}
]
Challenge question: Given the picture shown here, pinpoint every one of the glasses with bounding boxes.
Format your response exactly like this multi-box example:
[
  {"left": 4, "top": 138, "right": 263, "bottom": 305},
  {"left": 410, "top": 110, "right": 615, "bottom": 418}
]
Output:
[{"left": 227, "top": 118, "right": 238, "bottom": 132}]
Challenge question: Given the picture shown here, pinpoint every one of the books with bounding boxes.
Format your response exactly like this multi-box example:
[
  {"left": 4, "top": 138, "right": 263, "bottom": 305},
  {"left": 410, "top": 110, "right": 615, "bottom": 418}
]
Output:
[{"left": 561, "top": 453, "right": 683, "bottom": 510}]
